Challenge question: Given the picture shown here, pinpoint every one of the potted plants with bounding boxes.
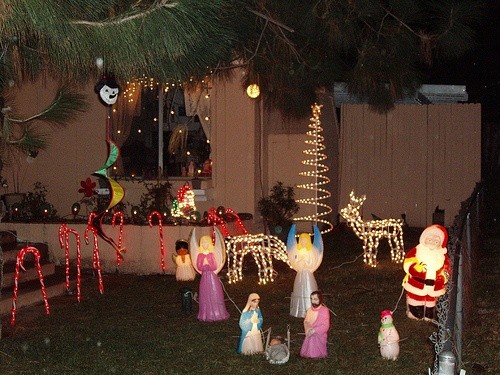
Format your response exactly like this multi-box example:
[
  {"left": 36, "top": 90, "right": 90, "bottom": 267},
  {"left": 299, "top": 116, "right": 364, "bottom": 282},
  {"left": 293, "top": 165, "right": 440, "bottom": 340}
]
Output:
[{"left": 258, "top": 181, "right": 300, "bottom": 241}]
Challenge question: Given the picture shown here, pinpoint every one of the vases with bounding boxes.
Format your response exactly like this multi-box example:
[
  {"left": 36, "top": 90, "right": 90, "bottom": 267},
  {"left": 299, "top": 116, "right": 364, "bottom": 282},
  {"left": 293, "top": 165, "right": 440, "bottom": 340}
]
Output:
[{"left": 4, "top": 193, "right": 25, "bottom": 209}]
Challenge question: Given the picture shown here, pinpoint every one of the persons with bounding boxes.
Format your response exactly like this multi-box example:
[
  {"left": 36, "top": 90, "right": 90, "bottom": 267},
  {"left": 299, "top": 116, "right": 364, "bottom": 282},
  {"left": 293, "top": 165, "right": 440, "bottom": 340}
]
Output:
[
  {"left": 300, "top": 291, "right": 329, "bottom": 356},
  {"left": 403, "top": 226, "right": 450, "bottom": 321},
  {"left": 173, "top": 239, "right": 196, "bottom": 281},
  {"left": 239, "top": 293, "right": 263, "bottom": 354},
  {"left": 287, "top": 224, "right": 323, "bottom": 317},
  {"left": 191, "top": 227, "right": 229, "bottom": 321},
  {"left": 266, "top": 338, "right": 290, "bottom": 364}
]
